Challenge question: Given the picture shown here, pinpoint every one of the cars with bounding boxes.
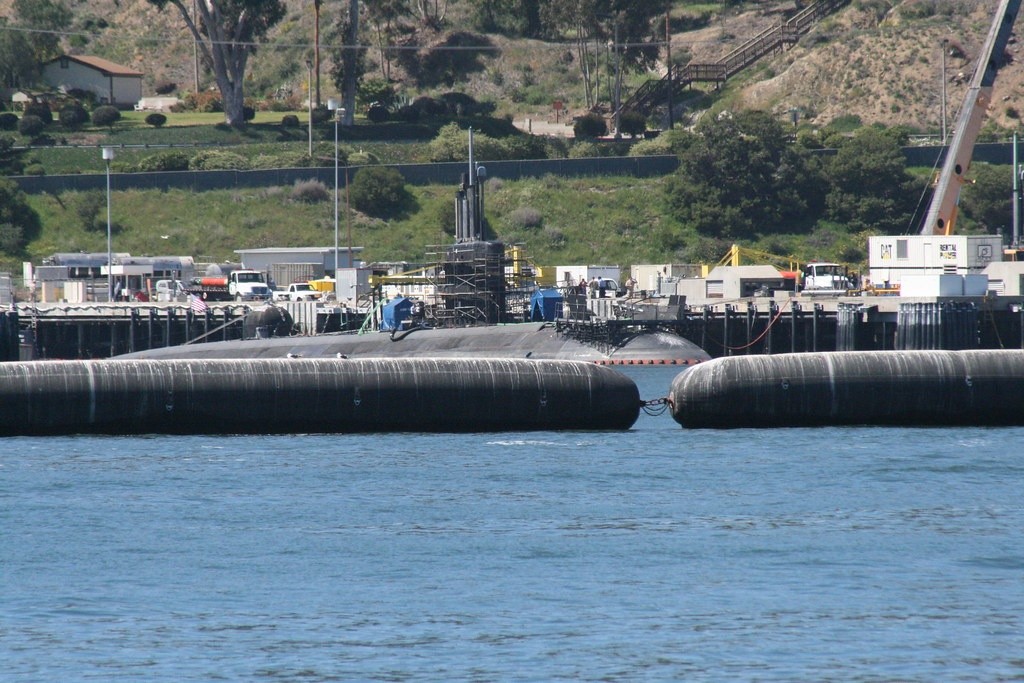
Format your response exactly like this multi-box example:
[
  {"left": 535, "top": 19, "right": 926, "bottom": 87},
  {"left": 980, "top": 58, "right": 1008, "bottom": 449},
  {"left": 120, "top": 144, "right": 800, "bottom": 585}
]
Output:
[{"left": 586, "top": 278, "right": 619, "bottom": 295}]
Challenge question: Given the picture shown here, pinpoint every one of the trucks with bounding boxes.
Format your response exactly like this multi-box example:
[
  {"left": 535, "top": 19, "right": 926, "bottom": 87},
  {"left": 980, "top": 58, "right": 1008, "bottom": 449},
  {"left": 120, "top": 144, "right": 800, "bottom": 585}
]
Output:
[
  {"left": 181, "top": 269, "right": 273, "bottom": 300},
  {"left": 800, "top": 261, "right": 854, "bottom": 291}
]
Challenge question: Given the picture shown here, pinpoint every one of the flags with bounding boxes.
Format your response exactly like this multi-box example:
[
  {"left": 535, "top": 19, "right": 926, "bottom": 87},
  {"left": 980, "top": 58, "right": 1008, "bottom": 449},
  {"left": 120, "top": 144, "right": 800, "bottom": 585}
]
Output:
[{"left": 190, "top": 293, "right": 208, "bottom": 313}]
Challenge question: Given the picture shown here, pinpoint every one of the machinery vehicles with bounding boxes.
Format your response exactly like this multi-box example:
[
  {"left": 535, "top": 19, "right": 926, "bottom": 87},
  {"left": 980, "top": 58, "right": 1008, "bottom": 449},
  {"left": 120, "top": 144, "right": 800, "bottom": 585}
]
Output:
[
  {"left": 367, "top": 244, "right": 558, "bottom": 292},
  {"left": 700, "top": 241, "right": 806, "bottom": 285},
  {"left": 896, "top": 0, "right": 1024, "bottom": 236}
]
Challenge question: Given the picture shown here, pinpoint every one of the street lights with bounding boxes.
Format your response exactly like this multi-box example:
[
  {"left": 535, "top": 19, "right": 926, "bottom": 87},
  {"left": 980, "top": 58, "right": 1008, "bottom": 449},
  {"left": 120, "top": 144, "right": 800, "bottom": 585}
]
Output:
[
  {"left": 305, "top": 59, "right": 315, "bottom": 157},
  {"left": 325, "top": 98, "right": 348, "bottom": 270},
  {"left": 942, "top": 36, "right": 949, "bottom": 142},
  {"left": 101, "top": 146, "right": 116, "bottom": 303}
]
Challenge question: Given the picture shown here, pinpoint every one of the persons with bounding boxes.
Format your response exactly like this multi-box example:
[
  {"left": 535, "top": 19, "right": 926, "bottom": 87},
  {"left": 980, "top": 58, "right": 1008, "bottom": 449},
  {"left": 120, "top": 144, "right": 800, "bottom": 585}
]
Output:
[
  {"left": 580, "top": 277, "right": 606, "bottom": 299},
  {"left": 26, "top": 280, "right": 39, "bottom": 302},
  {"left": 113, "top": 278, "right": 122, "bottom": 301},
  {"left": 625, "top": 277, "right": 637, "bottom": 298},
  {"left": 850, "top": 272, "right": 857, "bottom": 287}
]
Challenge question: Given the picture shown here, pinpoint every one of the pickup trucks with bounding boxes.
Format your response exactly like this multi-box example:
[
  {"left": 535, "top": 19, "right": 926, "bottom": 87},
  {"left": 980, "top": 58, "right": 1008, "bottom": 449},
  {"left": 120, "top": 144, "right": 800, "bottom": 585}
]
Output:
[{"left": 272, "top": 283, "right": 323, "bottom": 301}]
